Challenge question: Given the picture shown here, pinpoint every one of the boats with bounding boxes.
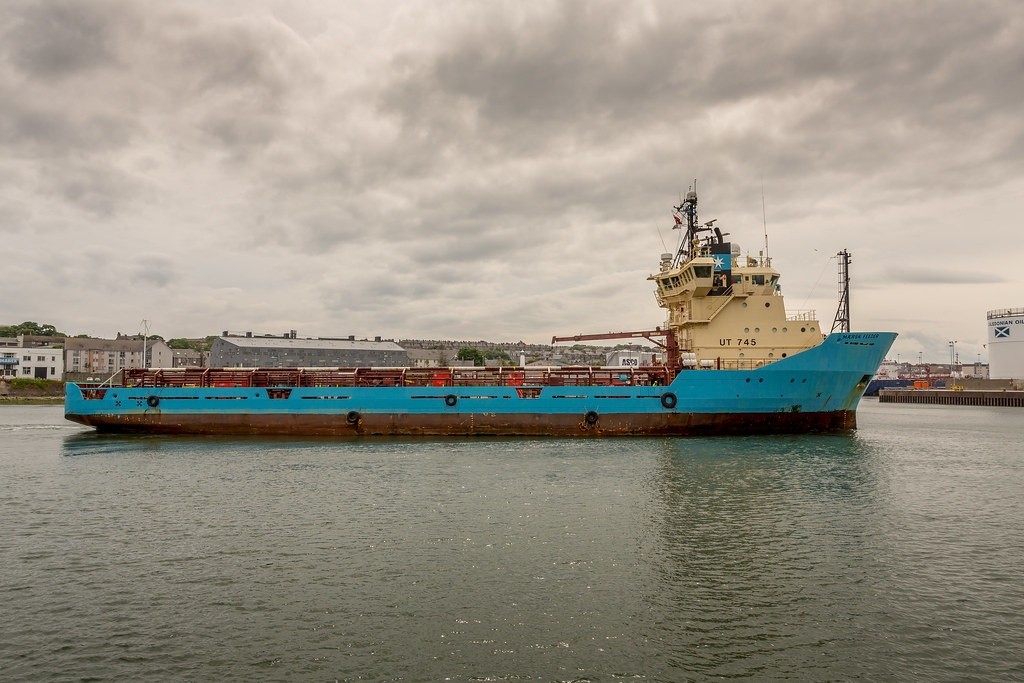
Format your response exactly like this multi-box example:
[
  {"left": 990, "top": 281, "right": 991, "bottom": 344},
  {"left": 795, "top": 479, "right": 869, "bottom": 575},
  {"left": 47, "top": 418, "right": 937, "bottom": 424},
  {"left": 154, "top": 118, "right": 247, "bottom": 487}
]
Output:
[
  {"left": 64, "top": 179, "right": 900, "bottom": 434},
  {"left": 862, "top": 357, "right": 946, "bottom": 397}
]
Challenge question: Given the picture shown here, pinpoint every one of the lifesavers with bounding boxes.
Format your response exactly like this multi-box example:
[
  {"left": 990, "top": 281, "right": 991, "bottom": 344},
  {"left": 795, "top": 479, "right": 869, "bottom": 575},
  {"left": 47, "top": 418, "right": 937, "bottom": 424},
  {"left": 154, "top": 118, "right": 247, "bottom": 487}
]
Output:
[
  {"left": 661, "top": 392, "right": 677, "bottom": 408},
  {"left": 585, "top": 411, "right": 599, "bottom": 423},
  {"left": 445, "top": 394, "right": 457, "bottom": 406},
  {"left": 147, "top": 395, "right": 160, "bottom": 408},
  {"left": 347, "top": 411, "right": 360, "bottom": 423}
]
